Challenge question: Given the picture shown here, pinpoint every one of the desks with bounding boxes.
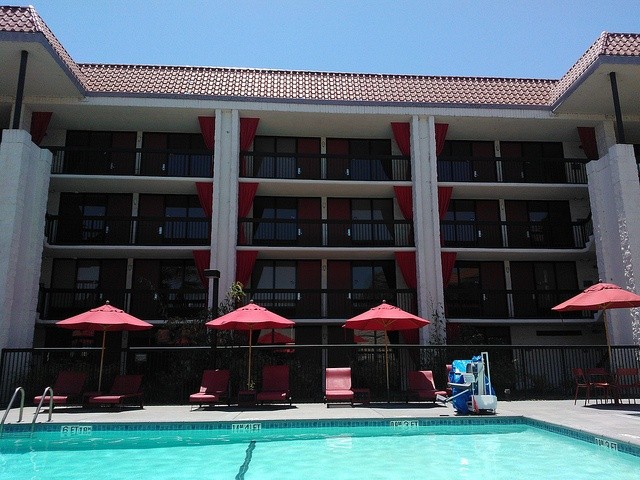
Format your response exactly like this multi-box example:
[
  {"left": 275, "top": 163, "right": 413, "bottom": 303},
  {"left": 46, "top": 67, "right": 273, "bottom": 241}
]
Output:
[
  {"left": 237, "top": 390, "right": 257, "bottom": 407},
  {"left": 79, "top": 393, "right": 100, "bottom": 407},
  {"left": 352, "top": 388, "right": 371, "bottom": 405}
]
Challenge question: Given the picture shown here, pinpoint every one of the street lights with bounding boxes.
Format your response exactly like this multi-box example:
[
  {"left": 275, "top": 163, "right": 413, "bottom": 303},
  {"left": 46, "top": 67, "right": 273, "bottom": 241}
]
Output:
[{"left": 204, "top": 270, "right": 220, "bottom": 369}]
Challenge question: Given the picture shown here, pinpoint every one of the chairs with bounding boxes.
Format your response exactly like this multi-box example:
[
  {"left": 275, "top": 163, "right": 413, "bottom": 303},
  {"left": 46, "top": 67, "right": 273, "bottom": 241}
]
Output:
[
  {"left": 189, "top": 368, "right": 229, "bottom": 412},
  {"left": 257, "top": 365, "right": 292, "bottom": 406},
  {"left": 587, "top": 368, "right": 610, "bottom": 404},
  {"left": 89, "top": 374, "right": 144, "bottom": 411},
  {"left": 609, "top": 366, "right": 640, "bottom": 408},
  {"left": 572, "top": 368, "right": 598, "bottom": 405},
  {"left": 406, "top": 370, "right": 447, "bottom": 406},
  {"left": 324, "top": 367, "right": 353, "bottom": 407},
  {"left": 34, "top": 370, "right": 76, "bottom": 413}
]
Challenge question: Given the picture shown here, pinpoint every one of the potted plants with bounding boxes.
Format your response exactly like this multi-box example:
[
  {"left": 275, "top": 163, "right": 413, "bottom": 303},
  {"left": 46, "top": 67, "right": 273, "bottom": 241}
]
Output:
[
  {"left": 157, "top": 316, "right": 178, "bottom": 343},
  {"left": 176, "top": 320, "right": 201, "bottom": 346}
]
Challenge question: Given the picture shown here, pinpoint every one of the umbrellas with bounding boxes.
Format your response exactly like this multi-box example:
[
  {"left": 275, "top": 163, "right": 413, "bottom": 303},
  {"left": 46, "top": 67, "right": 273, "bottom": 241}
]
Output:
[
  {"left": 258, "top": 331, "right": 294, "bottom": 346},
  {"left": 204, "top": 300, "right": 295, "bottom": 391},
  {"left": 342, "top": 300, "right": 431, "bottom": 404},
  {"left": 355, "top": 335, "right": 370, "bottom": 344},
  {"left": 56, "top": 301, "right": 154, "bottom": 395},
  {"left": 551, "top": 279, "right": 640, "bottom": 404}
]
